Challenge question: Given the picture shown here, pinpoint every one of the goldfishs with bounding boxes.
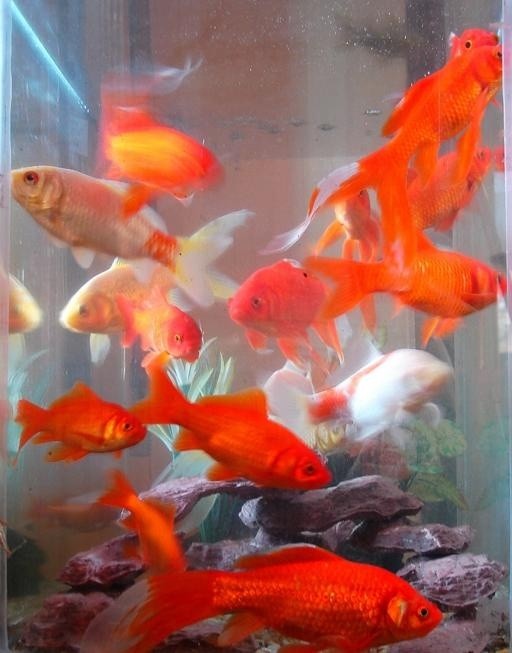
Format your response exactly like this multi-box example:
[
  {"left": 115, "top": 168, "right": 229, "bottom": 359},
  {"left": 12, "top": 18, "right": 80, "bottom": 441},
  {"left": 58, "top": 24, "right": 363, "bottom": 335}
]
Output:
[{"left": 0, "top": 28, "right": 512, "bottom": 653}]
